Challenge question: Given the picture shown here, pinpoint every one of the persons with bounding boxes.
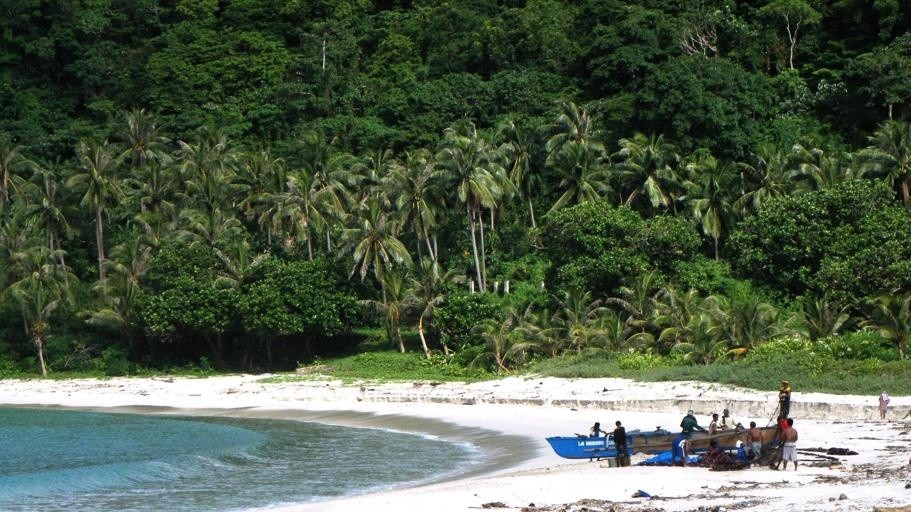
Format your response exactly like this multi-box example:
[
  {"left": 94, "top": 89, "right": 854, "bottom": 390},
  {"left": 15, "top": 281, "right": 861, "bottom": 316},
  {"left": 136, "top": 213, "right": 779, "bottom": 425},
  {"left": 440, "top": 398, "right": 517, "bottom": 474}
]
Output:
[
  {"left": 878, "top": 391, "right": 891, "bottom": 420},
  {"left": 613, "top": 421, "right": 627, "bottom": 467},
  {"left": 671, "top": 409, "right": 798, "bottom": 473},
  {"left": 588, "top": 421, "right": 609, "bottom": 462},
  {"left": 778, "top": 381, "right": 791, "bottom": 418}
]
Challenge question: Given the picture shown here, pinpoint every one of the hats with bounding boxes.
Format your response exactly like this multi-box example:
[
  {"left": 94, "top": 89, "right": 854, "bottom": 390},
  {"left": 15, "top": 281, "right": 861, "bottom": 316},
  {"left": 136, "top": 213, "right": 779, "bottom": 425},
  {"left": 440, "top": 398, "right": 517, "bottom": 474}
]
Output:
[{"left": 686, "top": 409, "right": 694, "bottom": 416}]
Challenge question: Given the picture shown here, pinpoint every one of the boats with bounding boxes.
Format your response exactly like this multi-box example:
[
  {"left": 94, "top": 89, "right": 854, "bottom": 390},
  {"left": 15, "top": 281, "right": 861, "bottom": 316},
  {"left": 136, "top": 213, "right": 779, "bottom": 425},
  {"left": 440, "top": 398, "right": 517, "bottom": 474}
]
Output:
[{"left": 545, "top": 423, "right": 789, "bottom": 459}]
